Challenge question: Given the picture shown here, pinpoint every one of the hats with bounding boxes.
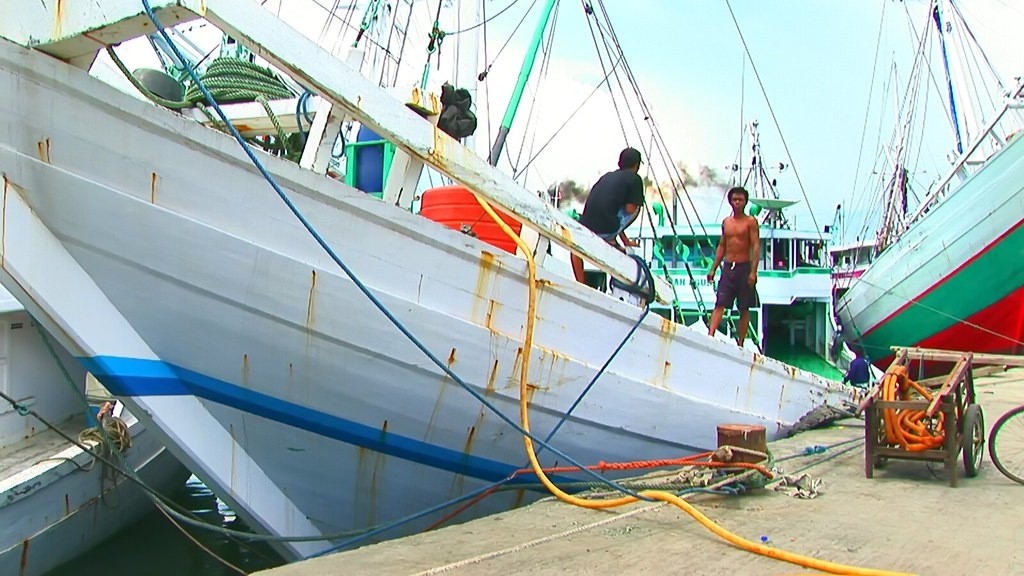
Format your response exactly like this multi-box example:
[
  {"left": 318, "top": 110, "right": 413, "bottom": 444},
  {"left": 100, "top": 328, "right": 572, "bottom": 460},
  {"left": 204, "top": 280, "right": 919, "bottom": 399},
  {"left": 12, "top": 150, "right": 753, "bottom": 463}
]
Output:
[{"left": 620, "top": 147, "right": 644, "bottom": 167}]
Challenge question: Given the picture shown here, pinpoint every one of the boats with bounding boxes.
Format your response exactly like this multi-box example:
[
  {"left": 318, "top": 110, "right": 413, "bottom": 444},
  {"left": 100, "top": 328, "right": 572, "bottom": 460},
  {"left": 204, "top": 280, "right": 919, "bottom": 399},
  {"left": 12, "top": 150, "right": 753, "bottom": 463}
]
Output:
[{"left": 0, "top": 0, "right": 1024, "bottom": 576}]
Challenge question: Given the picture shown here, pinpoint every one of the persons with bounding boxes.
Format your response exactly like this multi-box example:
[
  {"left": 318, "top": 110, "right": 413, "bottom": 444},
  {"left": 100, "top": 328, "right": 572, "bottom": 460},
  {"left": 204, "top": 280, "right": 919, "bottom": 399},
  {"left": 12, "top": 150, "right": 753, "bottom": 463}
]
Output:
[
  {"left": 842, "top": 348, "right": 869, "bottom": 390},
  {"left": 579, "top": 148, "right": 644, "bottom": 255},
  {"left": 707, "top": 187, "right": 760, "bottom": 347}
]
